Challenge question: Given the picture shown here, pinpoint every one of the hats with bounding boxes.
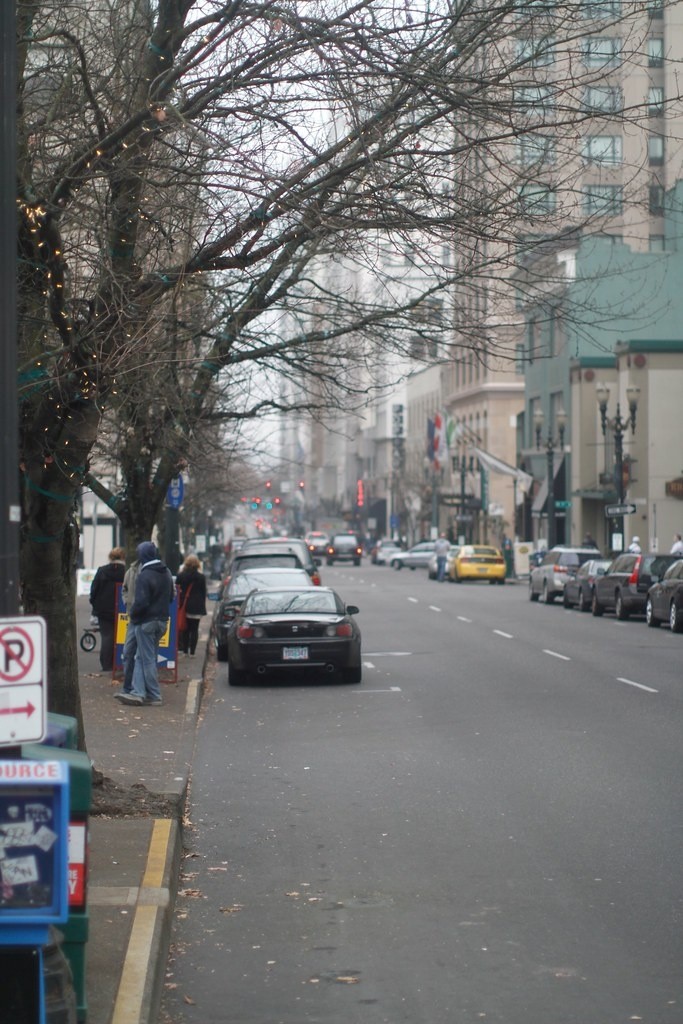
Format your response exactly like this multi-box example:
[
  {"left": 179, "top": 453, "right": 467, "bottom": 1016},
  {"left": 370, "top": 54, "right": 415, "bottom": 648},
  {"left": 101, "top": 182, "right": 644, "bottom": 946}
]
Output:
[{"left": 632, "top": 536, "right": 640, "bottom": 543}]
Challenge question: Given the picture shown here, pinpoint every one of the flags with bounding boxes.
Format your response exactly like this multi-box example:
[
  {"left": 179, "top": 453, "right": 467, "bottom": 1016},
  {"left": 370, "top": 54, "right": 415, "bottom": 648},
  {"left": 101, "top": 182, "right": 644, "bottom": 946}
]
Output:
[{"left": 421, "top": 411, "right": 449, "bottom": 471}]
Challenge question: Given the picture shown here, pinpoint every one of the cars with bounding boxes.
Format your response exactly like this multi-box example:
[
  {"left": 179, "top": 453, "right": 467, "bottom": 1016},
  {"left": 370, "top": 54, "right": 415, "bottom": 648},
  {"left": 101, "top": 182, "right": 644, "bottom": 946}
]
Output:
[
  {"left": 241, "top": 537, "right": 322, "bottom": 585},
  {"left": 449, "top": 545, "right": 507, "bottom": 585},
  {"left": 562, "top": 559, "right": 615, "bottom": 612},
  {"left": 207, "top": 568, "right": 315, "bottom": 662},
  {"left": 387, "top": 542, "right": 435, "bottom": 570},
  {"left": 426, "top": 546, "right": 458, "bottom": 580},
  {"left": 372, "top": 542, "right": 401, "bottom": 566},
  {"left": 645, "top": 559, "right": 683, "bottom": 633},
  {"left": 590, "top": 553, "right": 683, "bottom": 620},
  {"left": 223, "top": 586, "right": 364, "bottom": 687},
  {"left": 326, "top": 534, "right": 362, "bottom": 567},
  {"left": 304, "top": 532, "right": 330, "bottom": 556},
  {"left": 220, "top": 547, "right": 303, "bottom": 595},
  {"left": 529, "top": 546, "right": 604, "bottom": 604}
]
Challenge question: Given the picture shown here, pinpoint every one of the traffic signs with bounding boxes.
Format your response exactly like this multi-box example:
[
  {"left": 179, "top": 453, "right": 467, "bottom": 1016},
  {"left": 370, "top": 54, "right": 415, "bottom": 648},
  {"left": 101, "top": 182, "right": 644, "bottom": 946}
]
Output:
[{"left": 605, "top": 503, "right": 636, "bottom": 517}]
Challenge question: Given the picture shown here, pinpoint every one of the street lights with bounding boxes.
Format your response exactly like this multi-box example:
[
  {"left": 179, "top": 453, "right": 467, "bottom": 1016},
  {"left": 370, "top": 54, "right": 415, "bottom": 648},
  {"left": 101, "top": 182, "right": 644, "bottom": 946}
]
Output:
[
  {"left": 595, "top": 381, "right": 641, "bottom": 553},
  {"left": 422, "top": 455, "right": 447, "bottom": 542},
  {"left": 534, "top": 406, "right": 568, "bottom": 549},
  {"left": 382, "top": 469, "right": 402, "bottom": 540}
]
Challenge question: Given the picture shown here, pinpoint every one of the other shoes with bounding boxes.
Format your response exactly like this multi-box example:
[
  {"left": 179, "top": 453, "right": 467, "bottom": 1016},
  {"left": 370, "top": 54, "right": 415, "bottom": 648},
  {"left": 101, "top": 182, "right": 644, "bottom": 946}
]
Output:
[
  {"left": 142, "top": 699, "right": 163, "bottom": 706},
  {"left": 103, "top": 667, "right": 113, "bottom": 671},
  {"left": 114, "top": 689, "right": 131, "bottom": 698},
  {"left": 119, "top": 693, "right": 144, "bottom": 706},
  {"left": 182, "top": 653, "right": 197, "bottom": 658}
]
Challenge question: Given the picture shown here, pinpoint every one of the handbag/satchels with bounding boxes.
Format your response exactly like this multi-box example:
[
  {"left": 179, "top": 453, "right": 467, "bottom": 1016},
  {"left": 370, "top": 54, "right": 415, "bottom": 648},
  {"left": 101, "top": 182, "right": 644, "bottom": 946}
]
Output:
[
  {"left": 89, "top": 614, "right": 99, "bottom": 625},
  {"left": 178, "top": 608, "right": 188, "bottom": 630}
]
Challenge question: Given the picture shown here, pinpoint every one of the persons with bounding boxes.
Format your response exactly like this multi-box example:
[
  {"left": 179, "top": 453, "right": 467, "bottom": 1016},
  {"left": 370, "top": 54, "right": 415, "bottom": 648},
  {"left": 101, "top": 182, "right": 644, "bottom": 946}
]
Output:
[
  {"left": 501, "top": 533, "right": 512, "bottom": 578},
  {"left": 176, "top": 555, "right": 206, "bottom": 660},
  {"left": 670, "top": 533, "right": 683, "bottom": 557},
  {"left": 121, "top": 559, "right": 144, "bottom": 694},
  {"left": 89, "top": 546, "right": 127, "bottom": 671},
  {"left": 582, "top": 532, "right": 598, "bottom": 549},
  {"left": 112, "top": 541, "right": 174, "bottom": 706},
  {"left": 434, "top": 533, "right": 452, "bottom": 582},
  {"left": 629, "top": 536, "right": 642, "bottom": 554}
]
